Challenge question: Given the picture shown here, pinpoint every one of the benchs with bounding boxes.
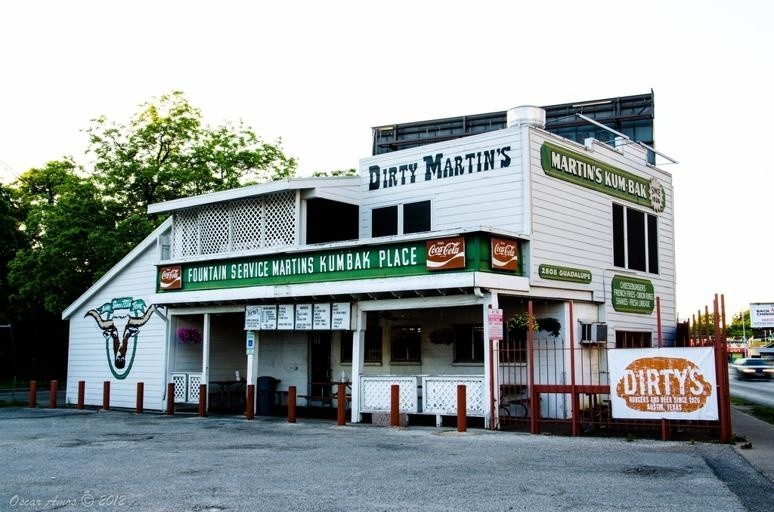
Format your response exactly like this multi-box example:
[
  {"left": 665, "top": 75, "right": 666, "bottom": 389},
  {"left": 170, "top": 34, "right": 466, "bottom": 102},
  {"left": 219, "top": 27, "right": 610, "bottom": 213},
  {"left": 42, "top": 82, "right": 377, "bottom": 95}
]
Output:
[
  {"left": 299, "top": 395, "right": 338, "bottom": 418},
  {"left": 508, "top": 397, "right": 542, "bottom": 419}
]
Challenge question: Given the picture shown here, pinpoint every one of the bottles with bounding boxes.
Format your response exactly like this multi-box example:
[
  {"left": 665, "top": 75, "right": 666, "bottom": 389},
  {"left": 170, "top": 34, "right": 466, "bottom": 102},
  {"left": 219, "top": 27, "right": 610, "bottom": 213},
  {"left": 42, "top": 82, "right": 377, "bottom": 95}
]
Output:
[
  {"left": 235, "top": 370, "right": 241, "bottom": 381},
  {"left": 340, "top": 368, "right": 348, "bottom": 383}
]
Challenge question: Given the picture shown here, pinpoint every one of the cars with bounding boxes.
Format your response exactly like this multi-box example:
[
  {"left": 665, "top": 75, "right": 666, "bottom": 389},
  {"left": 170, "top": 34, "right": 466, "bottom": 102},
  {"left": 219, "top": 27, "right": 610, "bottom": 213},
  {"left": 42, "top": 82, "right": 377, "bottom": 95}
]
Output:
[{"left": 729, "top": 358, "right": 773, "bottom": 383}]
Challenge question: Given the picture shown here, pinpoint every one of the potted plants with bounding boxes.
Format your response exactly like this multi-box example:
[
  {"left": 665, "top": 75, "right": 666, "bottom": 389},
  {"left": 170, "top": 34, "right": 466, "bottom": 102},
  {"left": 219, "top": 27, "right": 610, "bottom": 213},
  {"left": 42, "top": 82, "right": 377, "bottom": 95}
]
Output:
[{"left": 506, "top": 312, "right": 538, "bottom": 340}]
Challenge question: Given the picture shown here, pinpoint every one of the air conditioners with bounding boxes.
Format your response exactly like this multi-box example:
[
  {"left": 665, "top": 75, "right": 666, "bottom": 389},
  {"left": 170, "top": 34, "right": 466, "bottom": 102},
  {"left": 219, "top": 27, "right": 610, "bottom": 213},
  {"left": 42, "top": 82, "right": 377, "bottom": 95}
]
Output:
[{"left": 578, "top": 320, "right": 608, "bottom": 345}]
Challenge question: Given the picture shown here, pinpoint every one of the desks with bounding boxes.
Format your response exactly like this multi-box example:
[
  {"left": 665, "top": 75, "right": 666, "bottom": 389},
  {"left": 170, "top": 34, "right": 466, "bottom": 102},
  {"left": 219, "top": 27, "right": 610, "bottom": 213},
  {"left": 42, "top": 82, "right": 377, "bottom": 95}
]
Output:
[
  {"left": 309, "top": 381, "right": 352, "bottom": 418},
  {"left": 210, "top": 381, "right": 245, "bottom": 413},
  {"left": 501, "top": 385, "right": 527, "bottom": 416}
]
